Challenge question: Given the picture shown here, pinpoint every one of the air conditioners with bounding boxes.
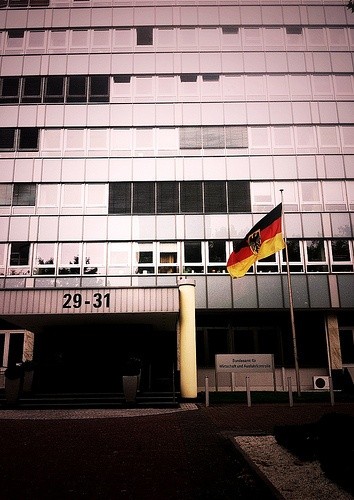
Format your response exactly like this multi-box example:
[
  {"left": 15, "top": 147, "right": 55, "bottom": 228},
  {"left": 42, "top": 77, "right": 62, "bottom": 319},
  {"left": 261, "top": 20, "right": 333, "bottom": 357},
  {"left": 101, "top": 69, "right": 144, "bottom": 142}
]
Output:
[{"left": 313, "top": 376, "right": 330, "bottom": 390}]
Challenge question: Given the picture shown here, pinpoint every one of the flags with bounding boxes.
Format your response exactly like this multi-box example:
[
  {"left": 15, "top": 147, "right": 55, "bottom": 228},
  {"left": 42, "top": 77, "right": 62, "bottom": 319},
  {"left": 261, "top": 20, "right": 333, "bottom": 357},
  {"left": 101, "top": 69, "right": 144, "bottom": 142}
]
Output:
[{"left": 226, "top": 202, "right": 285, "bottom": 280}]
